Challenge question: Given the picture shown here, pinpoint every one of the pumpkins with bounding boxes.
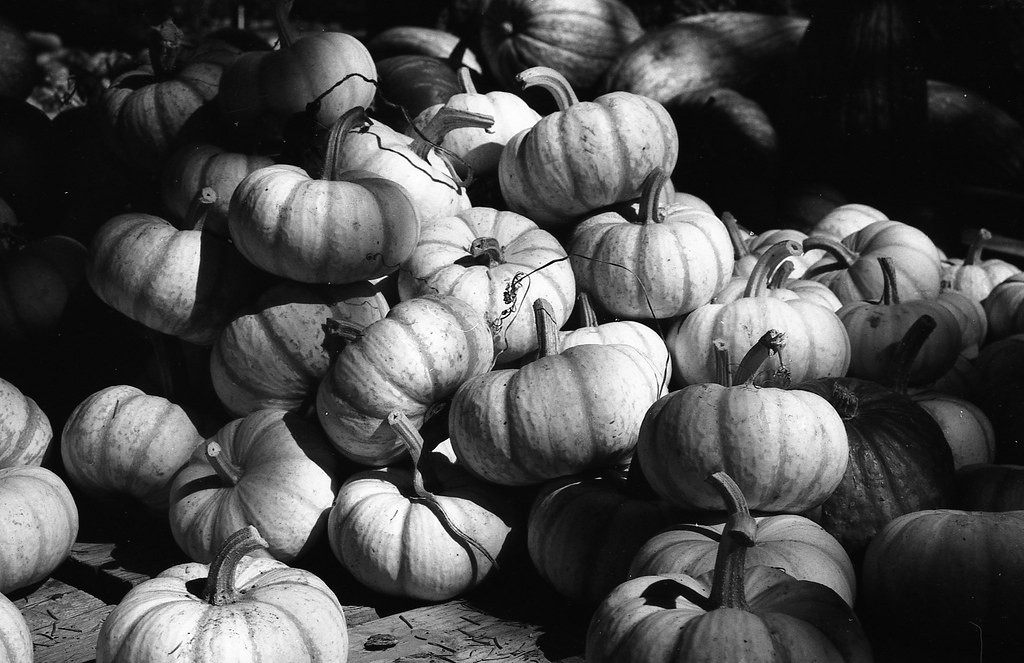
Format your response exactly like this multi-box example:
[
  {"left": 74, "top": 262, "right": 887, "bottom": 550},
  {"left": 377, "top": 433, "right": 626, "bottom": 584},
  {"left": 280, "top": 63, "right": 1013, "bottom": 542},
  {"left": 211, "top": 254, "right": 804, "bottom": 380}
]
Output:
[{"left": 0, "top": 0, "right": 1024, "bottom": 663}]
